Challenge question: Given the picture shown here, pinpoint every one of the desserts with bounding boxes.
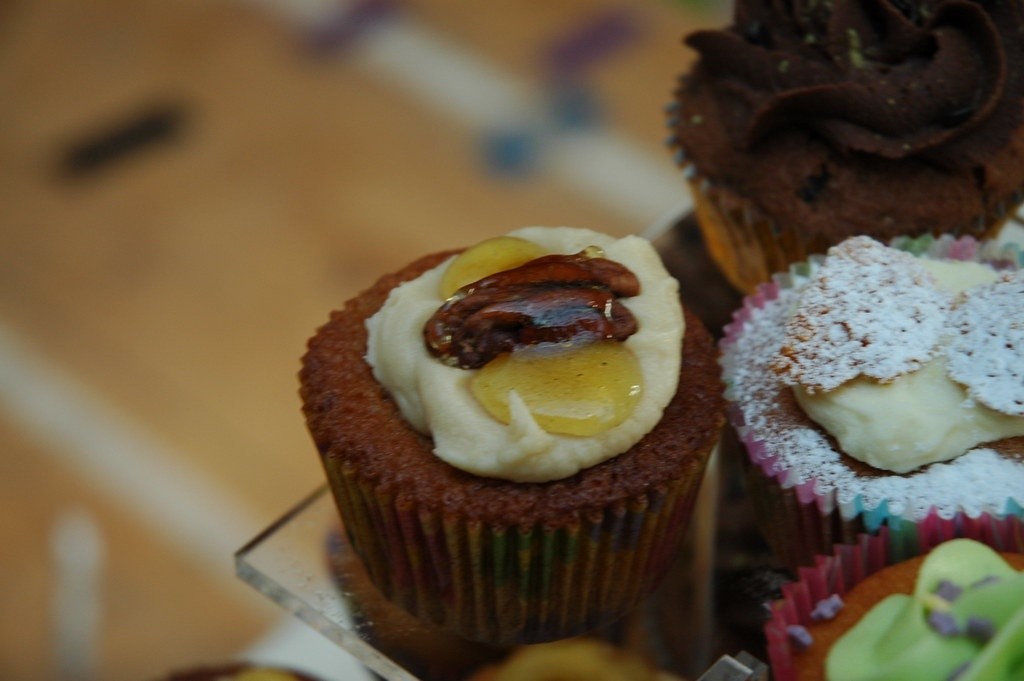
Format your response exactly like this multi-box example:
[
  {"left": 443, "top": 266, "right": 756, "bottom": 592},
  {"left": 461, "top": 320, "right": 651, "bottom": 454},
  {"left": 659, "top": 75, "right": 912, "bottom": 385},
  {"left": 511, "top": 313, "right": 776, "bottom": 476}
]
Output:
[{"left": 296, "top": 0, "right": 1024, "bottom": 681}]
